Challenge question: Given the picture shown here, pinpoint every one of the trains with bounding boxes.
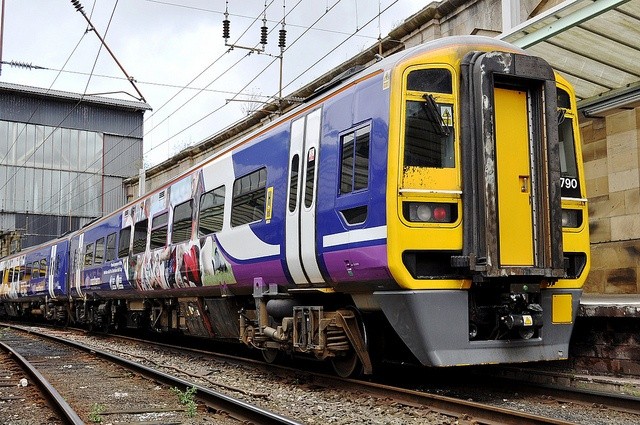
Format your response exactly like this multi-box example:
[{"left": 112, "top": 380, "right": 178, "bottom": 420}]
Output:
[{"left": 2, "top": 35, "right": 592, "bottom": 384}]
